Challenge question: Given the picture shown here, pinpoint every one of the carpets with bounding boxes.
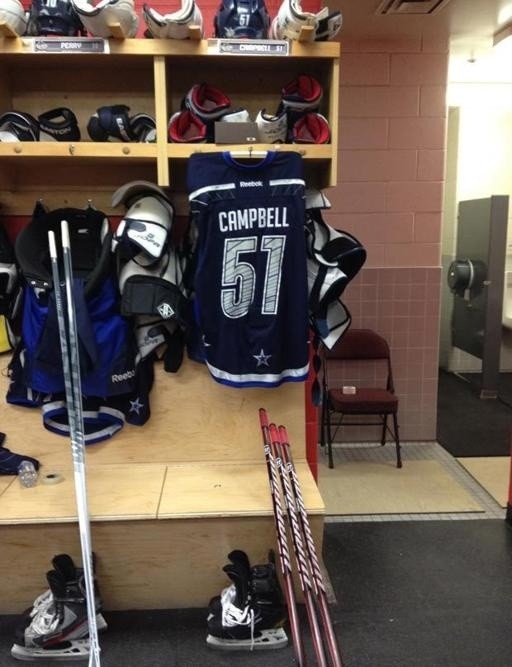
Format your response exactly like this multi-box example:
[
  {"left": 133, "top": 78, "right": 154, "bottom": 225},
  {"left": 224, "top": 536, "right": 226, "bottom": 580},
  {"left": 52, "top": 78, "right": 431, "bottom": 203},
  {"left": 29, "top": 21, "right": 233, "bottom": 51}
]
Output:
[{"left": 1, "top": 519, "right": 512, "bottom": 666}]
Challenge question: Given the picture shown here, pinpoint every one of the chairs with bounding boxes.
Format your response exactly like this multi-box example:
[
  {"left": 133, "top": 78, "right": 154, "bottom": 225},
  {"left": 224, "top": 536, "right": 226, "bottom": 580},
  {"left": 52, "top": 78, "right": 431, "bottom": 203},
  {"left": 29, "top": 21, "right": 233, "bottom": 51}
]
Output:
[{"left": 317, "top": 330, "right": 402, "bottom": 469}]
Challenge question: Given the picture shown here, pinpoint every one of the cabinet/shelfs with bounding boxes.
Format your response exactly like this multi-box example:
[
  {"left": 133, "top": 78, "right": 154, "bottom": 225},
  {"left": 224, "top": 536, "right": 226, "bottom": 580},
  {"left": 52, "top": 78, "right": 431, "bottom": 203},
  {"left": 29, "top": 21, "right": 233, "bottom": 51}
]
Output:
[
  {"left": 2, "top": 355, "right": 325, "bottom": 615},
  {"left": 2, "top": 36, "right": 340, "bottom": 192}
]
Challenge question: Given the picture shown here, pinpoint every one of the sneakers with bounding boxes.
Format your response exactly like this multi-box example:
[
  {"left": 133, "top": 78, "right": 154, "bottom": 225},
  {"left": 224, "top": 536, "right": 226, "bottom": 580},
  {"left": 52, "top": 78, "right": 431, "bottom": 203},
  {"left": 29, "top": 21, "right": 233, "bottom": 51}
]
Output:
[
  {"left": 208, "top": 549, "right": 283, "bottom": 614},
  {"left": 11, "top": 569, "right": 107, "bottom": 647},
  {"left": 207, "top": 561, "right": 290, "bottom": 643},
  {"left": 22, "top": 552, "right": 96, "bottom": 621}
]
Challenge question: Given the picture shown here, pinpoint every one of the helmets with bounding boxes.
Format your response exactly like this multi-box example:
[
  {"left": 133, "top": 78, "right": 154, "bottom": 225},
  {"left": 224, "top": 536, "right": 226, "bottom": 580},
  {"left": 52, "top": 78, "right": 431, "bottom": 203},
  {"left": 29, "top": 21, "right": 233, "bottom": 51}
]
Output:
[
  {"left": 213, "top": 0, "right": 271, "bottom": 40},
  {"left": 30, "top": 0, "right": 81, "bottom": 37}
]
